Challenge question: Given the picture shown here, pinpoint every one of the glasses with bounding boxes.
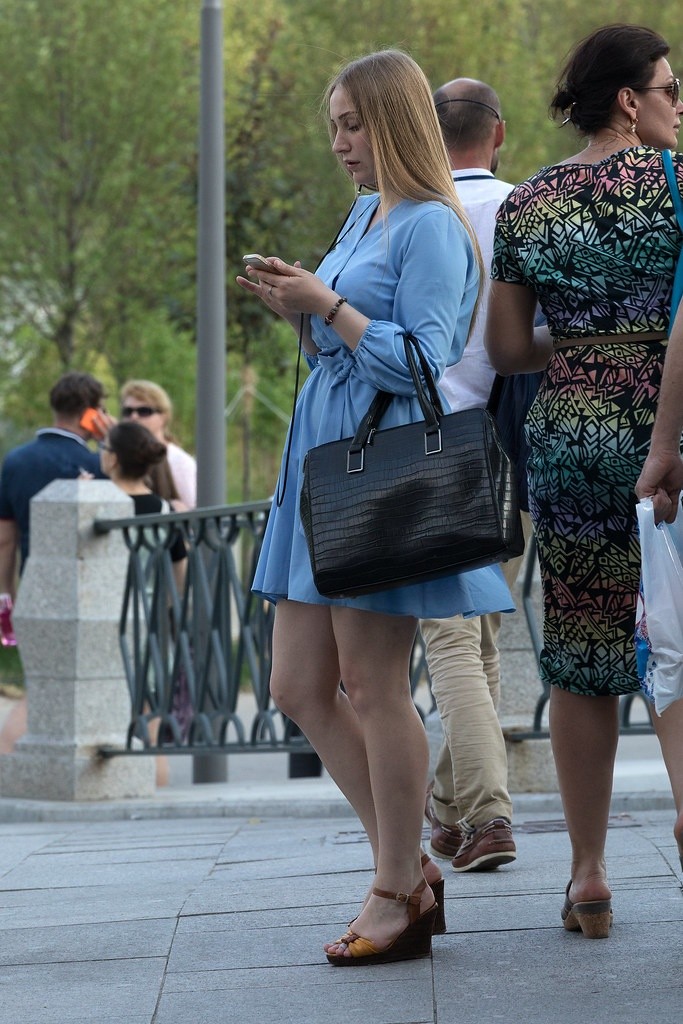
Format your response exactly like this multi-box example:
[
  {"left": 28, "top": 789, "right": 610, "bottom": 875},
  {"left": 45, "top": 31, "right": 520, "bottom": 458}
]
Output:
[
  {"left": 634, "top": 78, "right": 680, "bottom": 107},
  {"left": 120, "top": 406, "right": 157, "bottom": 418},
  {"left": 98, "top": 437, "right": 113, "bottom": 454}
]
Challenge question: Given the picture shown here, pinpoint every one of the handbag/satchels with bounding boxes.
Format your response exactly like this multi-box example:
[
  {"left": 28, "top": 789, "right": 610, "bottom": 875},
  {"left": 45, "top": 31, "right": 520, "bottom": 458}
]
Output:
[
  {"left": 633, "top": 495, "right": 683, "bottom": 714},
  {"left": 299, "top": 333, "right": 525, "bottom": 600}
]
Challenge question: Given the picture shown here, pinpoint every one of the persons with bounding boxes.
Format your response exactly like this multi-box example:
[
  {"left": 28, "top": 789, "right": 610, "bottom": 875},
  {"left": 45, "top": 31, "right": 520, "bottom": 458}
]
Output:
[
  {"left": 236, "top": 49, "right": 516, "bottom": 968},
  {"left": 0, "top": 376, "right": 197, "bottom": 754},
  {"left": 484, "top": 26, "right": 683, "bottom": 937},
  {"left": 636, "top": 299, "right": 683, "bottom": 524},
  {"left": 423, "top": 78, "right": 515, "bottom": 872}
]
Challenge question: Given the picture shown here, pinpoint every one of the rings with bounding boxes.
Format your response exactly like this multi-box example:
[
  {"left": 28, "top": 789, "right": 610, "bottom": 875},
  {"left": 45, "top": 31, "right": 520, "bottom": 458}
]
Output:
[{"left": 268, "top": 286, "right": 273, "bottom": 296}]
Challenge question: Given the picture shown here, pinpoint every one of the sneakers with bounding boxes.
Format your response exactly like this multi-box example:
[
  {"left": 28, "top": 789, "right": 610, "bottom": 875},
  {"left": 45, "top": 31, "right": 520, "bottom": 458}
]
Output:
[
  {"left": 451, "top": 818, "right": 517, "bottom": 873},
  {"left": 425, "top": 779, "right": 466, "bottom": 859}
]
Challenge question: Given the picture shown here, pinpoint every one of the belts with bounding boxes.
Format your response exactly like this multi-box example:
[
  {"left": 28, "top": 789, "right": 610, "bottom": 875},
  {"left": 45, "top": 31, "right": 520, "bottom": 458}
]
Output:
[{"left": 554, "top": 332, "right": 669, "bottom": 351}]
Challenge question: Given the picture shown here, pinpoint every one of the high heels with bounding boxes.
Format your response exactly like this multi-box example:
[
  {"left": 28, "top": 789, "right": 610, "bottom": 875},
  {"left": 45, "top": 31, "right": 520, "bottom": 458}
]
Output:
[
  {"left": 562, "top": 878, "right": 613, "bottom": 938},
  {"left": 347, "top": 854, "right": 448, "bottom": 935},
  {"left": 325, "top": 879, "right": 439, "bottom": 968}
]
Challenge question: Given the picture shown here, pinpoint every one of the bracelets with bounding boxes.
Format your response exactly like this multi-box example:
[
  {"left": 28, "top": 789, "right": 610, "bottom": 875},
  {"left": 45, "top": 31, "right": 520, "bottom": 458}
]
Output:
[{"left": 325, "top": 297, "right": 347, "bottom": 325}]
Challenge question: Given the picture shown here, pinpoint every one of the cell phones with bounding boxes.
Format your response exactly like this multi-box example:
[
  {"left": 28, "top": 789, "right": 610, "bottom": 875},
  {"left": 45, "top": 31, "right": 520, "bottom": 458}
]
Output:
[
  {"left": 243, "top": 254, "right": 281, "bottom": 275},
  {"left": 81, "top": 408, "right": 107, "bottom": 436}
]
